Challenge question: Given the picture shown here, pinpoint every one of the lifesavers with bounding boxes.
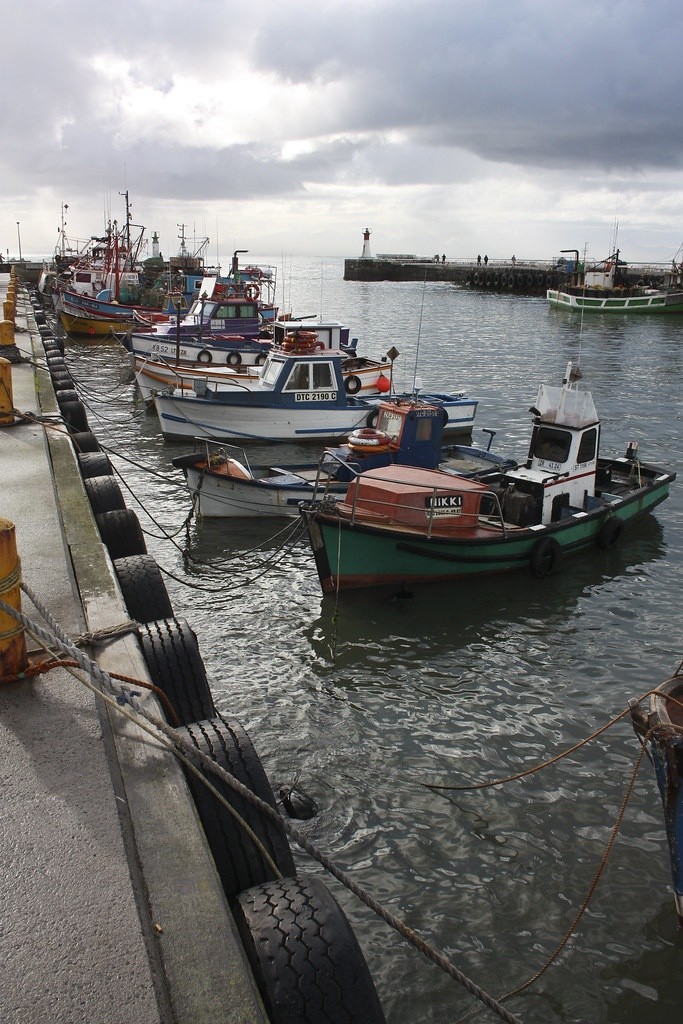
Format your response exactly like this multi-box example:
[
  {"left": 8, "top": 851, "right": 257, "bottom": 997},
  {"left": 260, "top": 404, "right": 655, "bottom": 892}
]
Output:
[
  {"left": 232, "top": 873, "right": 388, "bottom": 1023},
  {"left": 113, "top": 553, "right": 175, "bottom": 624},
  {"left": 367, "top": 409, "right": 379, "bottom": 429},
  {"left": 84, "top": 475, "right": 127, "bottom": 515},
  {"left": 225, "top": 284, "right": 260, "bottom": 301},
  {"left": 139, "top": 615, "right": 216, "bottom": 730},
  {"left": 530, "top": 536, "right": 563, "bottom": 577},
  {"left": 31, "top": 292, "right": 114, "bottom": 480},
  {"left": 197, "top": 350, "right": 267, "bottom": 365},
  {"left": 439, "top": 407, "right": 448, "bottom": 427},
  {"left": 96, "top": 509, "right": 148, "bottom": 561},
  {"left": 282, "top": 331, "right": 319, "bottom": 354},
  {"left": 347, "top": 427, "right": 391, "bottom": 452},
  {"left": 344, "top": 375, "right": 361, "bottom": 394},
  {"left": 596, "top": 516, "right": 625, "bottom": 549},
  {"left": 92, "top": 280, "right": 104, "bottom": 291},
  {"left": 175, "top": 715, "right": 298, "bottom": 901},
  {"left": 250, "top": 268, "right": 263, "bottom": 280}
]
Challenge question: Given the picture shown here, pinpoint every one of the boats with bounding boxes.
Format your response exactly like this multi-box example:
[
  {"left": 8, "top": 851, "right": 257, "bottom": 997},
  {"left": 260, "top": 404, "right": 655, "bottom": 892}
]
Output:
[
  {"left": 148, "top": 266, "right": 478, "bottom": 444},
  {"left": 546, "top": 221, "right": 683, "bottom": 315},
  {"left": 297, "top": 242, "right": 676, "bottom": 596},
  {"left": 172, "top": 269, "right": 520, "bottom": 521},
  {"left": 626, "top": 653, "right": 683, "bottom": 923},
  {"left": 38, "top": 188, "right": 399, "bottom": 417}
]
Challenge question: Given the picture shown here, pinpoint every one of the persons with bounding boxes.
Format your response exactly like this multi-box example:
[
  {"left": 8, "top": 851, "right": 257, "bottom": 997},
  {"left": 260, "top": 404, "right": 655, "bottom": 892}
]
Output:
[
  {"left": 511, "top": 255, "right": 516, "bottom": 266},
  {"left": 0, "top": 253, "right": 5, "bottom": 263},
  {"left": 442, "top": 254, "right": 446, "bottom": 264},
  {"left": 478, "top": 255, "right": 481, "bottom": 266},
  {"left": 436, "top": 254, "right": 440, "bottom": 264},
  {"left": 484, "top": 255, "right": 488, "bottom": 265}
]
